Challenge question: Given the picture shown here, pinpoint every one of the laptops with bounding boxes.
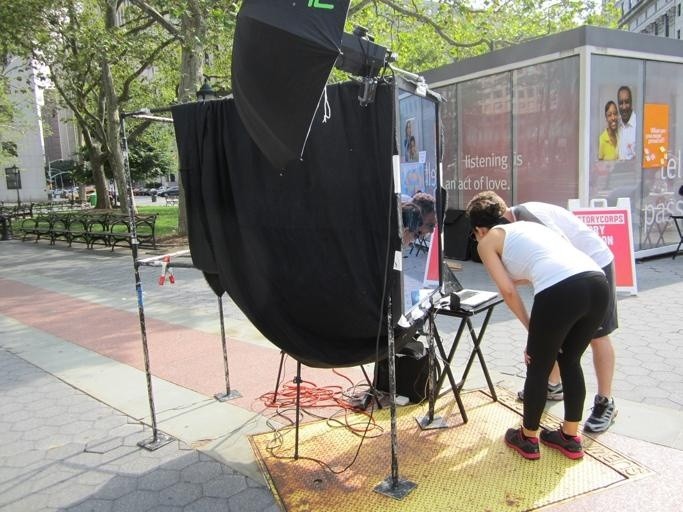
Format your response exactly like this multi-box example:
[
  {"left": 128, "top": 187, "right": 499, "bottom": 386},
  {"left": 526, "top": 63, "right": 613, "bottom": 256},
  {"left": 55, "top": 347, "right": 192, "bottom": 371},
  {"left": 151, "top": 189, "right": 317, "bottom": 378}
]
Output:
[
  {"left": 443, "top": 261, "right": 498, "bottom": 307},
  {"left": 419, "top": 289, "right": 434, "bottom": 300}
]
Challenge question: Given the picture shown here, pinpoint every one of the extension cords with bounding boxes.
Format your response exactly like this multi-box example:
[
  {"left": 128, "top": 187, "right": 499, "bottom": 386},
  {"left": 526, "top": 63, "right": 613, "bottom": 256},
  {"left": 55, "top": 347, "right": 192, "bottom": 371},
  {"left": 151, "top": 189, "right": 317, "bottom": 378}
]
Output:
[{"left": 360, "top": 389, "right": 371, "bottom": 410}]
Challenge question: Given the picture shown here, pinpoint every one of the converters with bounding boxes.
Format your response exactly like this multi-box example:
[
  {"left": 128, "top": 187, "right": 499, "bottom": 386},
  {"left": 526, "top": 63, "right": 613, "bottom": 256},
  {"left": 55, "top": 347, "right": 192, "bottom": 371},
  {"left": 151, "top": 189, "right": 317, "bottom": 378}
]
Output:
[{"left": 395, "top": 396, "right": 410, "bottom": 406}]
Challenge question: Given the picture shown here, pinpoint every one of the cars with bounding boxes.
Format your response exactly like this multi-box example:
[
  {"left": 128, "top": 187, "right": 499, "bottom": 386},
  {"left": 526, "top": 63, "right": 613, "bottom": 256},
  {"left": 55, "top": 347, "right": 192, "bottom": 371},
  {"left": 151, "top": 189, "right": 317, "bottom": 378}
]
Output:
[{"left": 50, "top": 185, "right": 180, "bottom": 201}]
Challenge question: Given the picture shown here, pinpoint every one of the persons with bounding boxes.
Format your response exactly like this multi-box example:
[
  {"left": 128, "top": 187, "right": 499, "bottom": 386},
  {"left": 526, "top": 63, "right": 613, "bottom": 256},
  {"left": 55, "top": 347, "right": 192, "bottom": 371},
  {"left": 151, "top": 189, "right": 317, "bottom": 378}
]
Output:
[
  {"left": 407, "top": 136, "right": 419, "bottom": 162},
  {"left": 394, "top": 201, "right": 425, "bottom": 250},
  {"left": 406, "top": 190, "right": 439, "bottom": 235},
  {"left": 463, "top": 192, "right": 619, "bottom": 435},
  {"left": 597, "top": 100, "right": 619, "bottom": 162},
  {"left": 402, "top": 121, "right": 412, "bottom": 161},
  {"left": 464, "top": 196, "right": 611, "bottom": 461},
  {"left": 613, "top": 85, "right": 636, "bottom": 161}
]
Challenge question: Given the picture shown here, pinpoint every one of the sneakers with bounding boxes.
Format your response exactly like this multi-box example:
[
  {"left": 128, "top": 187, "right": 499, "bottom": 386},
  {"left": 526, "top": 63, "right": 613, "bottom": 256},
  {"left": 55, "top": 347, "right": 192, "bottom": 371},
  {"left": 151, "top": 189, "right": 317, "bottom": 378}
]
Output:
[
  {"left": 538, "top": 422, "right": 584, "bottom": 460},
  {"left": 503, "top": 424, "right": 540, "bottom": 460},
  {"left": 517, "top": 381, "right": 563, "bottom": 402},
  {"left": 583, "top": 395, "right": 618, "bottom": 433}
]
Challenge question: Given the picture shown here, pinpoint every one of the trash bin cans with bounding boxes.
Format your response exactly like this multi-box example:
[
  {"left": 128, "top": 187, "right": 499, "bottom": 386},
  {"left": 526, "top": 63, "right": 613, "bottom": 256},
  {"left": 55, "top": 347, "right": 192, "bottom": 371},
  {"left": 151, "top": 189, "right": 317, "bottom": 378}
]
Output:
[
  {"left": 152, "top": 195, "right": 155, "bottom": 202},
  {"left": 47, "top": 194, "right": 51, "bottom": 200},
  {"left": 89, "top": 195, "right": 95, "bottom": 205}
]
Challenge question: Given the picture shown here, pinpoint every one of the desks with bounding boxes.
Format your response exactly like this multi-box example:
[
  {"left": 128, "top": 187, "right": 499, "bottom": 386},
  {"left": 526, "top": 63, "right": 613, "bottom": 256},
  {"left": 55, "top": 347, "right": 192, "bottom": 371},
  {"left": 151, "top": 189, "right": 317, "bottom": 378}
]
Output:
[{"left": 429, "top": 288, "right": 503, "bottom": 423}]
